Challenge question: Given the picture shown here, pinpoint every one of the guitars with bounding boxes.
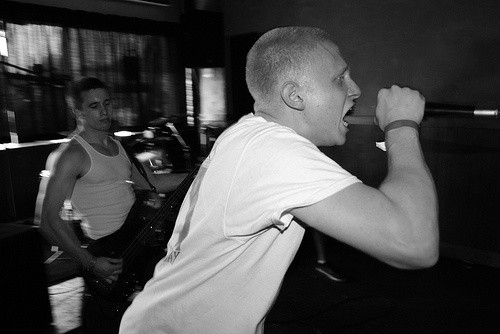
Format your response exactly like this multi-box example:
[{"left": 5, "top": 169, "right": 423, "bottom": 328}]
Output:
[{"left": 49, "top": 156, "right": 206, "bottom": 334}]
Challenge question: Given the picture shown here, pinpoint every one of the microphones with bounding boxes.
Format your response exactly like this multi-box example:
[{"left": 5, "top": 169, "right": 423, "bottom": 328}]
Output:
[
  {"left": 130, "top": 153, "right": 148, "bottom": 180},
  {"left": 423, "top": 102, "right": 500, "bottom": 120}
]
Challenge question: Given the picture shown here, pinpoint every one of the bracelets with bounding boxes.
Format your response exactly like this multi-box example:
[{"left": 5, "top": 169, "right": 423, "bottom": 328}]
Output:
[{"left": 379, "top": 119, "right": 420, "bottom": 132}]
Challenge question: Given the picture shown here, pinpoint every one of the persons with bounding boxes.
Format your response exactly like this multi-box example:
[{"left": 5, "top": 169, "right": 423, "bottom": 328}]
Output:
[
  {"left": 35, "top": 77, "right": 179, "bottom": 303},
  {"left": 117, "top": 25, "right": 440, "bottom": 334}
]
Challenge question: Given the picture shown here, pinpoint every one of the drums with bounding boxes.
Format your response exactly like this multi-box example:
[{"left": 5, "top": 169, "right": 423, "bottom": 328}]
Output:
[{"left": 128, "top": 137, "right": 183, "bottom": 175}]
